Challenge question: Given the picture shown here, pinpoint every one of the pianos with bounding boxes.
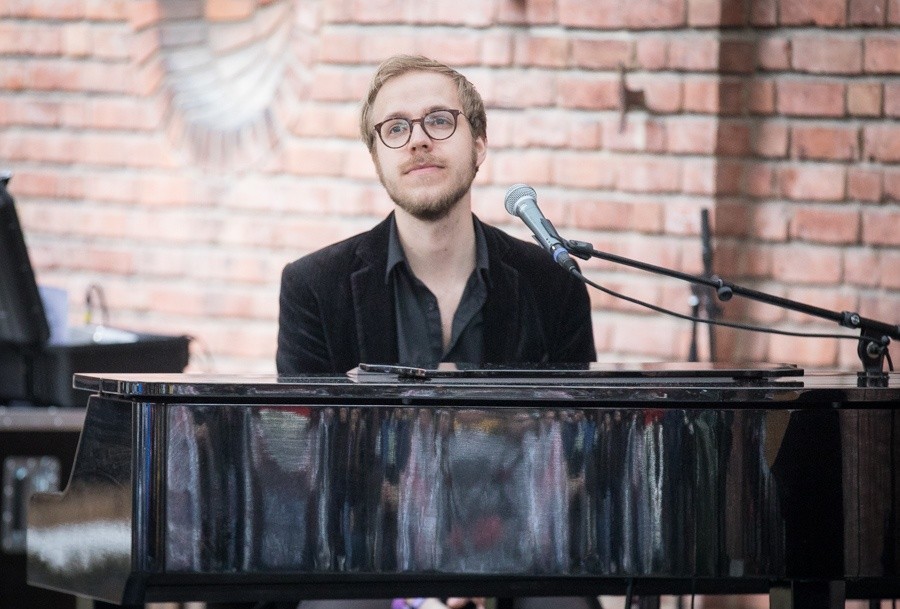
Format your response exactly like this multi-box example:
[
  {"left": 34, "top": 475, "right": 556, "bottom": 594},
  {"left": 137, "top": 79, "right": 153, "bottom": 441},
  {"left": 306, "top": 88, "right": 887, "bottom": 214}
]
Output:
[
  {"left": 27, "top": 363, "right": 900, "bottom": 609},
  {"left": 0, "top": 177, "right": 197, "bottom": 609}
]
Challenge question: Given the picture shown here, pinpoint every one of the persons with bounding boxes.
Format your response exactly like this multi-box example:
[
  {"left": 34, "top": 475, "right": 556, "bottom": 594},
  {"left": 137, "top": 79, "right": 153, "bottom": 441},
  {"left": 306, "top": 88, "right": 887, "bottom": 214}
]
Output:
[{"left": 275, "top": 54, "right": 599, "bottom": 379}]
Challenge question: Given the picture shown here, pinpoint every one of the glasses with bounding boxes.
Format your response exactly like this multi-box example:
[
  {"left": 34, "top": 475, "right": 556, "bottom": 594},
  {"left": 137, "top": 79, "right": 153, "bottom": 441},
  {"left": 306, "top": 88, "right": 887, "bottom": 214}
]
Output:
[{"left": 372, "top": 109, "right": 472, "bottom": 149}]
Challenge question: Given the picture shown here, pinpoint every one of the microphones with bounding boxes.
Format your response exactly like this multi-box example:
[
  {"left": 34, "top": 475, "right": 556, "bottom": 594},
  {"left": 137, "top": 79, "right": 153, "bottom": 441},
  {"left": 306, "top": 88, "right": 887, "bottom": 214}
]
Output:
[{"left": 502, "top": 183, "right": 579, "bottom": 275}]
